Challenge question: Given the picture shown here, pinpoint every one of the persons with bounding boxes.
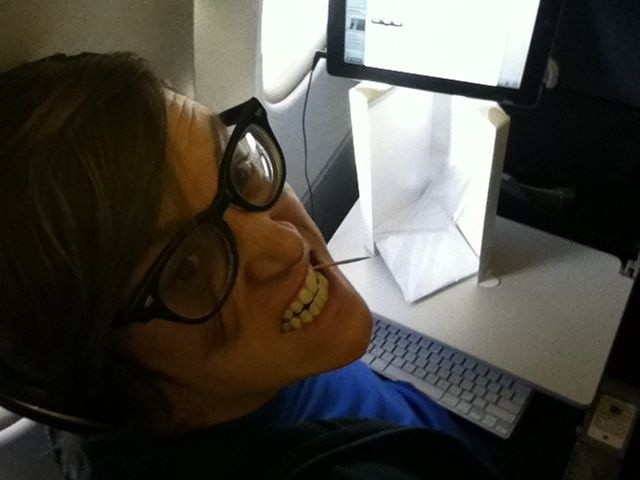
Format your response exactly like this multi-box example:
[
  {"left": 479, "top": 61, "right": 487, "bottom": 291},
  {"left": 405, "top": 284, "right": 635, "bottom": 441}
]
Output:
[{"left": 1, "top": 52, "right": 500, "bottom": 479}]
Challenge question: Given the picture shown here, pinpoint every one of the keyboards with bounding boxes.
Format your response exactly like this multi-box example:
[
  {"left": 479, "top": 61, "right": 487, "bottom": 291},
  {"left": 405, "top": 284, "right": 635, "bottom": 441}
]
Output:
[{"left": 359, "top": 314, "right": 534, "bottom": 440}]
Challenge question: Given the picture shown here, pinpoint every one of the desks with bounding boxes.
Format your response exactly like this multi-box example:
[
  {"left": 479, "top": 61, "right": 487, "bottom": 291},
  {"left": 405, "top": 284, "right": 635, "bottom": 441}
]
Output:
[{"left": 320, "top": 187, "right": 635, "bottom": 409}]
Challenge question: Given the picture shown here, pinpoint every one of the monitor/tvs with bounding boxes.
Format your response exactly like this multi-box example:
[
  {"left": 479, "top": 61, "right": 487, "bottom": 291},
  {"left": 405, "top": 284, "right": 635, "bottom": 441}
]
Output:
[{"left": 323, "top": 0, "right": 561, "bottom": 108}]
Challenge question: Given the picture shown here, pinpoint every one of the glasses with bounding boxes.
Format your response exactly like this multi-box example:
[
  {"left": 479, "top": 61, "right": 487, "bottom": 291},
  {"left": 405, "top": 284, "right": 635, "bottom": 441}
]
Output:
[{"left": 103, "top": 95, "right": 287, "bottom": 329}]
